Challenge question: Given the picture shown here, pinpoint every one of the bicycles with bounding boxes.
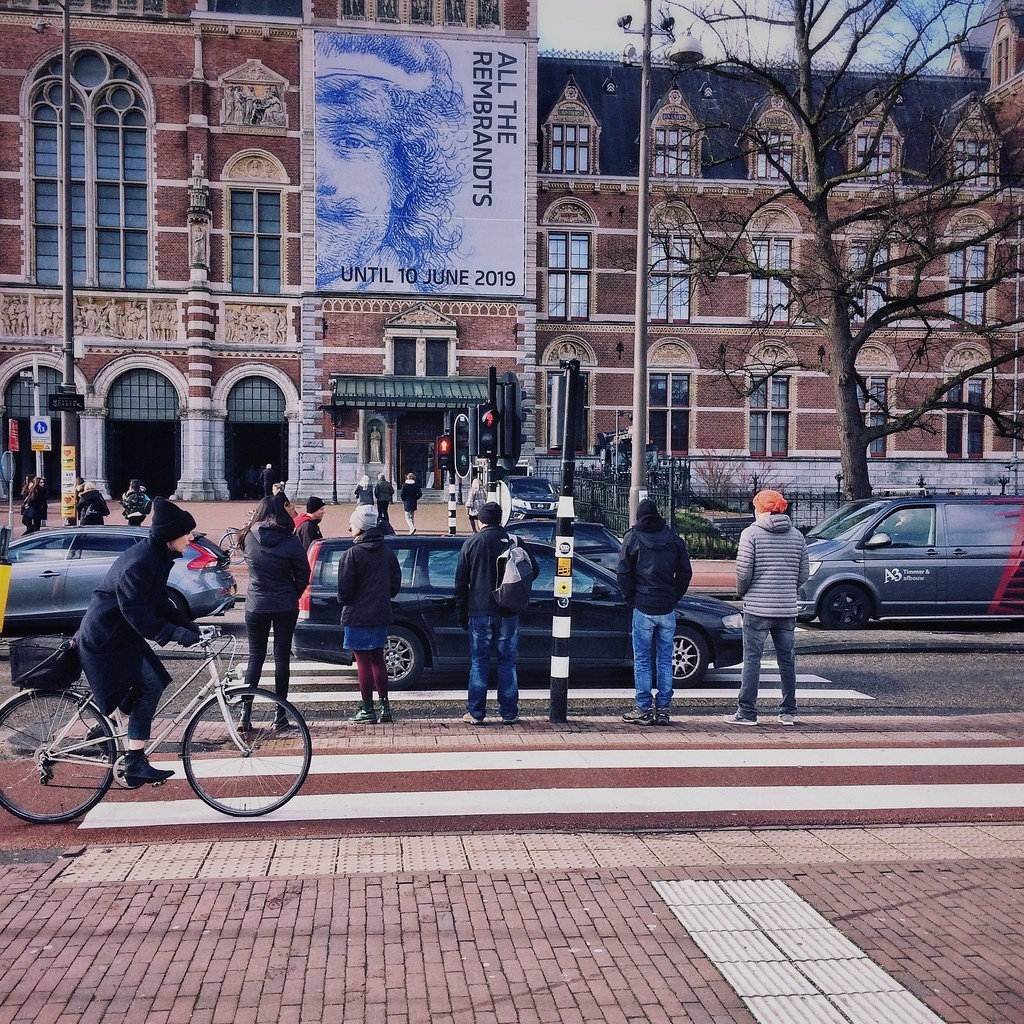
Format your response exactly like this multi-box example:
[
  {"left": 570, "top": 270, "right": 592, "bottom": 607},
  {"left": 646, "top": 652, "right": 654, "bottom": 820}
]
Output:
[
  {"left": 218, "top": 509, "right": 258, "bottom": 566},
  {"left": 0, "top": 625, "right": 312, "bottom": 824}
]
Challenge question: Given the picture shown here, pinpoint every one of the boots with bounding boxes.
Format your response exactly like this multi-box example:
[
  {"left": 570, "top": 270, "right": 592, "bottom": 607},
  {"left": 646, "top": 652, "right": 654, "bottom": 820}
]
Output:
[
  {"left": 272, "top": 704, "right": 289, "bottom": 730},
  {"left": 347, "top": 700, "right": 377, "bottom": 724},
  {"left": 124, "top": 751, "right": 175, "bottom": 786},
  {"left": 86, "top": 717, "right": 118, "bottom": 756},
  {"left": 377, "top": 697, "right": 393, "bottom": 724},
  {"left": 237, "top": 701, "right": 253, "bottom": 731}
]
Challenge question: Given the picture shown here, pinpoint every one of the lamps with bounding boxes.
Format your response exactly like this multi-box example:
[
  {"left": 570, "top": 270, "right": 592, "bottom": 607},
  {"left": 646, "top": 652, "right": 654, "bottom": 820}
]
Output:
[
  {"left": 916, "top": 346, "right": 924, "bottom": 365},
  {"left": 718, "top": 343, "right": 726, "bottom": 362},
  {"left": 616, "top": 341, "right": 624, "bottom": 360},
  {"left": 818, "top": 345, "right": 826, "bottom": 363}
]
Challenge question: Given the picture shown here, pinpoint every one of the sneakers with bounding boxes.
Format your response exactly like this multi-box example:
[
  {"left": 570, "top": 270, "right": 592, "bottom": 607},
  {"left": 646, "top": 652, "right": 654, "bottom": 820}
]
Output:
[
  {"left": 503, "top": 714, "right": 519, "bottom": 724},
  {"left": 655, "top": 707, "right": 669, "bottom": 725},
  {"left": 723, "top": 712, "right": 758, "bottom": 725},
  {"left": 778, "top": 714, "right": 794, "bottom": 725},
  {"left": 463, "top": 712, "right": 483, "bottom": 724},
  {"left": 622, "top": 707, "right": 654, "bottom": 726}
]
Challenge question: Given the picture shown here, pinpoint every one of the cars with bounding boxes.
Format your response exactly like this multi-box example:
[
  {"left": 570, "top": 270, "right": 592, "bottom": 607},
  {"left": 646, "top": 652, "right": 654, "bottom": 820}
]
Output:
[
  {"left": 496, "top": 521, "right": 627, "bottom": 572},
  {"left": 4, "top": 526, "right": 238, "bottom": 635},
  {"left": 504, "top": 473, "right": 562, "bottom": 520}
]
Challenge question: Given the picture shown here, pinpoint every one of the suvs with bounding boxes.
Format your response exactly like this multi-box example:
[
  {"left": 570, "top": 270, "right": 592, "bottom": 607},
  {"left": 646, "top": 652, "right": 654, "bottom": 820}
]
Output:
[{"left": 291, "top": 534, "right": 744, "bottom": 689}]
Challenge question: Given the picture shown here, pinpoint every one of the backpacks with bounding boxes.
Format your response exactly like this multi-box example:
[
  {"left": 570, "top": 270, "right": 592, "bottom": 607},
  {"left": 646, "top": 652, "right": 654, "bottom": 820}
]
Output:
[
  {"left": 140, "top": 494, "right": 152, "bottom": 514},
  {"left": 492, "top": 532, "right": 533, "bottom": 611}
]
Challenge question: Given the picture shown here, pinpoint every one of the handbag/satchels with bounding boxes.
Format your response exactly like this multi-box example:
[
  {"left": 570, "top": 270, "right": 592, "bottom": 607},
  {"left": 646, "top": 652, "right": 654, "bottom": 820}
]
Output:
[
  {"left": 88, "top": 496, "right": 110, "bottom": 517},
  {"left": 7, "top": 634, "right": 82, "bottom": 690},
  {"left": 284, "top": 501, "right": 298, "bottom": 519}
]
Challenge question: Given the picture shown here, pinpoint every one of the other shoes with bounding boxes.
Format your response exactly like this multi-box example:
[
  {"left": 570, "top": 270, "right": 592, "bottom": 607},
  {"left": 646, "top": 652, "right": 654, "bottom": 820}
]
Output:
[{"left": 409, "top": 527, "right": 416, "bottom": 535}]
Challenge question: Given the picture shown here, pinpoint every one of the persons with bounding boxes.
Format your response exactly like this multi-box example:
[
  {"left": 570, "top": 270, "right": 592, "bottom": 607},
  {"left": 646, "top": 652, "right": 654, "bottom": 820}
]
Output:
[
  {"left": 454, "top": 501, "right": 540, "bottom": 722},
  {"left": 246, "top": 464, "right": 265, "bottom": 500},
  {"left": 238, "top": 495, "right": 311, "bottom": 732},
  {"left": 355, "top": 475, "right": 374, "bottom": 505},
  {"left": 401, "top": 473, "right": 422, "bottom": 534},
  {"left": 77, "top": 496, "right": 202, "bottom": 786},
  {"left": 272, "top": 483, "right": 291, "bottom": 507},
  {"left": 337, "top": 504, "right": 402, "bottom": 723},
  {"left": 725, "top": 489, "right": 809, "bottom": 725},
  {"left": 375, "top": 473, "right": 394, "bottom": 520},
  {"left": 259, "top": 464, "right": 276, "bottom": 497},
  {"left": 616, "top": 499, "right": 692, "bottom": 723},
  {"left": 0, "top": 297, "right": 63, "bottom": 337},
  {"left": 226, "top": 304, "right": 286, "bottom": 345},
  {"left": 76, "top": 477, "right": 104, "bottom": 525},
  {"left": 72, "top": 296, "right": 178, "bottom": 341},
  {"left": 122, "top": 479, "right": 146, "bottom": 526},
  {"left": 465, "top": 478, "right": 487, "bottom": 533},
  {"left": 21, "top": 475, "right": 47, "bottom": 533},
  {"left": 293, "top": 497, "right": 326, "bottom": 549}
]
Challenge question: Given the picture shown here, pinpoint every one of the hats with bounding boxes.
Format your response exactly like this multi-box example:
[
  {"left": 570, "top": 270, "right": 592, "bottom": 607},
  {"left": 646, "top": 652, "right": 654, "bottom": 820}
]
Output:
[
  {"left": 29, "top": 474, "right": 35, "bottom": 479},
  {"left": 478, "top": 501, "right": 502, "bottom": 525},
  {"left": 350, "top": 504, "right": 378, "bottom": 530},
  {"left": 149, "top": 496, "right": 197, "bottom": 542},
  {"left": 306, "top": 497, "right": 325, "bottom": 514},
  {"left": 131, "top": 480, "right": 141, "bottom": 488},
  {"left": 753, "top": 490, "right": 787, "bottom": 513},
  {"left": 637, "top": 499, "right": 658, "bottom": 517}
]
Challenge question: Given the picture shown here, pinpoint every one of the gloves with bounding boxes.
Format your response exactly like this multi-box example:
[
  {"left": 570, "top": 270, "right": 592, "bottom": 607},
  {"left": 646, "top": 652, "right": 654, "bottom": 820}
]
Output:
[
  {"left": 180, "top": 630, "right": 200, "bottom": 647},
  {"left": 459, "top": 624, "right": 469, "bottom": 631},
  {"left": 194, "top": 626, "right": 209, "bottom": 635}
]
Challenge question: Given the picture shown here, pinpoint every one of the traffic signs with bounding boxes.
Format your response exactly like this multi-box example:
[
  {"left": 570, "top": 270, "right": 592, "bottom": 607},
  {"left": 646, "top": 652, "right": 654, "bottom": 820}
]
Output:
[{"left": 9, "top": 419, "right": 20, "bottom": 451}]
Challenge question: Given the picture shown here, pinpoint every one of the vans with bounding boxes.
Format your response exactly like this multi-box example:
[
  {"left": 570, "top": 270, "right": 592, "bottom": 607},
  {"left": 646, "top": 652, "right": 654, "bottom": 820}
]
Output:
[{"left": 795, "top": 494, "right": 1024, "bottom": 630}]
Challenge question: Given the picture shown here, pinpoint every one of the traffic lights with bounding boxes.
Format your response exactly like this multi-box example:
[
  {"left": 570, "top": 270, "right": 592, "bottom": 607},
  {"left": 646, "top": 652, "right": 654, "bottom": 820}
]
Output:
[
  {"left": 454, "top": 414, "right": 470, "bottom": 479},
  {"left": 437, "top": 436, "right": 453, "bottom": 473},
  {"left": 478, "top": 403, "right": 501, "bottom": 457},
  {"left": 500, "top": 371, "right": 528, "bottom": 470}
]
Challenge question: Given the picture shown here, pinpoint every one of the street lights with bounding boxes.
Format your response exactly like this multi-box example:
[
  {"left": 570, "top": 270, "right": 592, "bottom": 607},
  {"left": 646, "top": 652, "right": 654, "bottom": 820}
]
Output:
[{"left": 616, "top": 13, "right": 677, "bottom": 528}]
